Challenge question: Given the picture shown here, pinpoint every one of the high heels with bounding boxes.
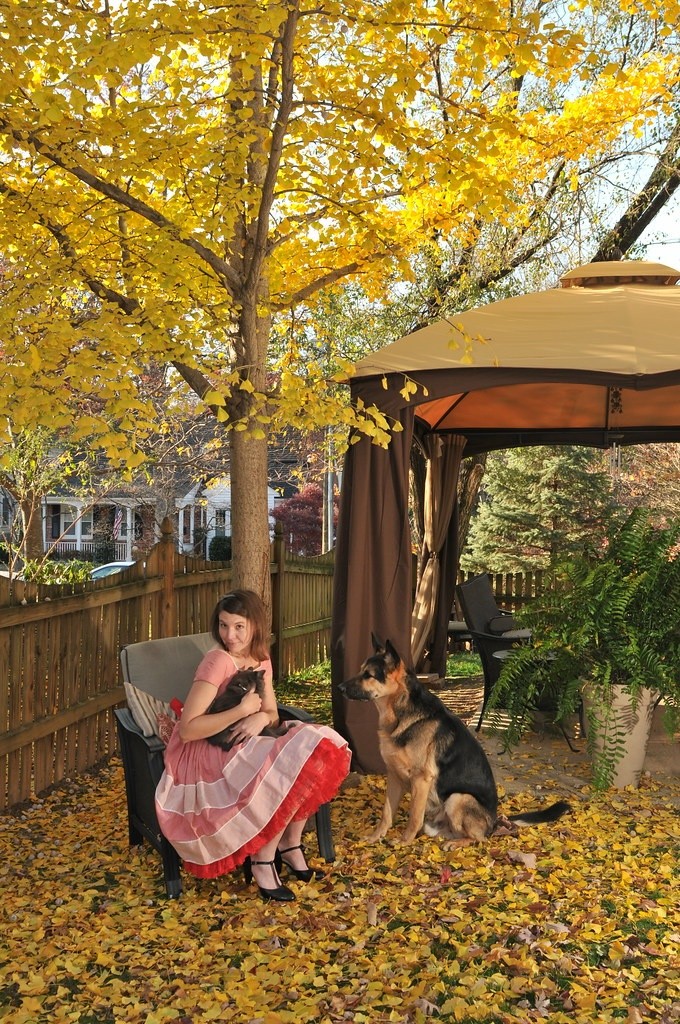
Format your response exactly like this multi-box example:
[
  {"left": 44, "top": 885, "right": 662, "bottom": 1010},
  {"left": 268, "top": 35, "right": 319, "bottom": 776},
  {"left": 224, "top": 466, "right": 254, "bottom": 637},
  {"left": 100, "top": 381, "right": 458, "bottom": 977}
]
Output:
[
  {"left": 243, "top": 843, "right": 296, "bottom": 901},
  {"left": 274, "top": 843, "right": 326, "bottom": 882}
]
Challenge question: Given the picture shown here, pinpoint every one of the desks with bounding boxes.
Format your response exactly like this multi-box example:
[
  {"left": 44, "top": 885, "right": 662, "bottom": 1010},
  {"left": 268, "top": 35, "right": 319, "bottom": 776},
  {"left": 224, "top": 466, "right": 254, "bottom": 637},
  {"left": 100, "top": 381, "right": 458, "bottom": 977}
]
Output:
[{"left": 492, "top": 649, "right": 581, "bottom": 756}]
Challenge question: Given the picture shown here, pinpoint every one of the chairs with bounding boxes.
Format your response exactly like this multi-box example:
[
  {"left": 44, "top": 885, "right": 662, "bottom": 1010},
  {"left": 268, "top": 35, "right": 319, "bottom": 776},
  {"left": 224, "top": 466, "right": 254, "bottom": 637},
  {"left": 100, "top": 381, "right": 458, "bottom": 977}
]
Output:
[
  {"left": 111, "top": 632, "right": 336, "bottom": 900},
  {"left": 455, "top": 572, "right": 586, "bottom": 738}
]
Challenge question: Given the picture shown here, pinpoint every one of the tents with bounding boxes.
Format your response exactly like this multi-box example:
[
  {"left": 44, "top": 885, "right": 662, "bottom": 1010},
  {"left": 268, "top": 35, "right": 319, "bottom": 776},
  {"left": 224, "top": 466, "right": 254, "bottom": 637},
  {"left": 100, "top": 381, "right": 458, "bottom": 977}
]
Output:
[{"left": 328, "top": 260, "right": 680, "bottom": 774}]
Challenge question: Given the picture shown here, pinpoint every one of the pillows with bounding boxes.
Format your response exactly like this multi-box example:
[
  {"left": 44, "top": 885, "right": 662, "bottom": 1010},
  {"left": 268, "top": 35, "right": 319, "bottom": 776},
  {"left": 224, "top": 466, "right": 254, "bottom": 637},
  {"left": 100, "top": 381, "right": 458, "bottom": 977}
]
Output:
[{"left": 124, "top": 682, "right": 180, "bottom": 738}]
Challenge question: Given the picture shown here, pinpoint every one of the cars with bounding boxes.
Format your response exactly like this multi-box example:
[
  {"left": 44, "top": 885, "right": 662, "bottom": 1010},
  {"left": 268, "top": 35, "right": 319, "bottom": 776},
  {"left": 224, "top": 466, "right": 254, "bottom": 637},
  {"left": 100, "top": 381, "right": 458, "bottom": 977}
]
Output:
[{"left": 88, "top": 561, "right": 145, "bottom": 581}]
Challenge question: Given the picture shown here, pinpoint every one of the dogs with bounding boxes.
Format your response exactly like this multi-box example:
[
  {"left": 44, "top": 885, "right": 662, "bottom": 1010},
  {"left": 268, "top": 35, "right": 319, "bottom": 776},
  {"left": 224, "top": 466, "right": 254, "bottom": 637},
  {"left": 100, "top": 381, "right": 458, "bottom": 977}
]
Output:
[{"left": 338, "top": 632, "right": 570, "bottom": 850}]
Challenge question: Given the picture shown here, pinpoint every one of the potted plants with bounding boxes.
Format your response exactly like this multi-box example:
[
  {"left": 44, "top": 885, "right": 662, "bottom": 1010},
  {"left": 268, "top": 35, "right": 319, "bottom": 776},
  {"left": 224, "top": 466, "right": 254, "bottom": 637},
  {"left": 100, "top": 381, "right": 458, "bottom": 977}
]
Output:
[{"left": 483, "top": 493, "right": 679, "bottom": 803}]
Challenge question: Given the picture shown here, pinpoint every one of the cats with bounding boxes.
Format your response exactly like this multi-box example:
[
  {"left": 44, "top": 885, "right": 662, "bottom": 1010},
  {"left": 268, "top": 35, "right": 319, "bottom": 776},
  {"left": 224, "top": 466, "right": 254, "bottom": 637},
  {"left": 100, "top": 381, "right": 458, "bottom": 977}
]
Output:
[{"left": 205, "top": 666, "right": 296, "bottom": 752}]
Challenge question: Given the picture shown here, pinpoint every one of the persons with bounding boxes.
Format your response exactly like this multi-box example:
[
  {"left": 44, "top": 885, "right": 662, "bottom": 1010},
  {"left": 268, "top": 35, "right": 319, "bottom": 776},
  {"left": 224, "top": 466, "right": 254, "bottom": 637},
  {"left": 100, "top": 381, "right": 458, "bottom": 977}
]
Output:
[{"left": 154, "top": 590, "right": 353, "bottom": 901}]
